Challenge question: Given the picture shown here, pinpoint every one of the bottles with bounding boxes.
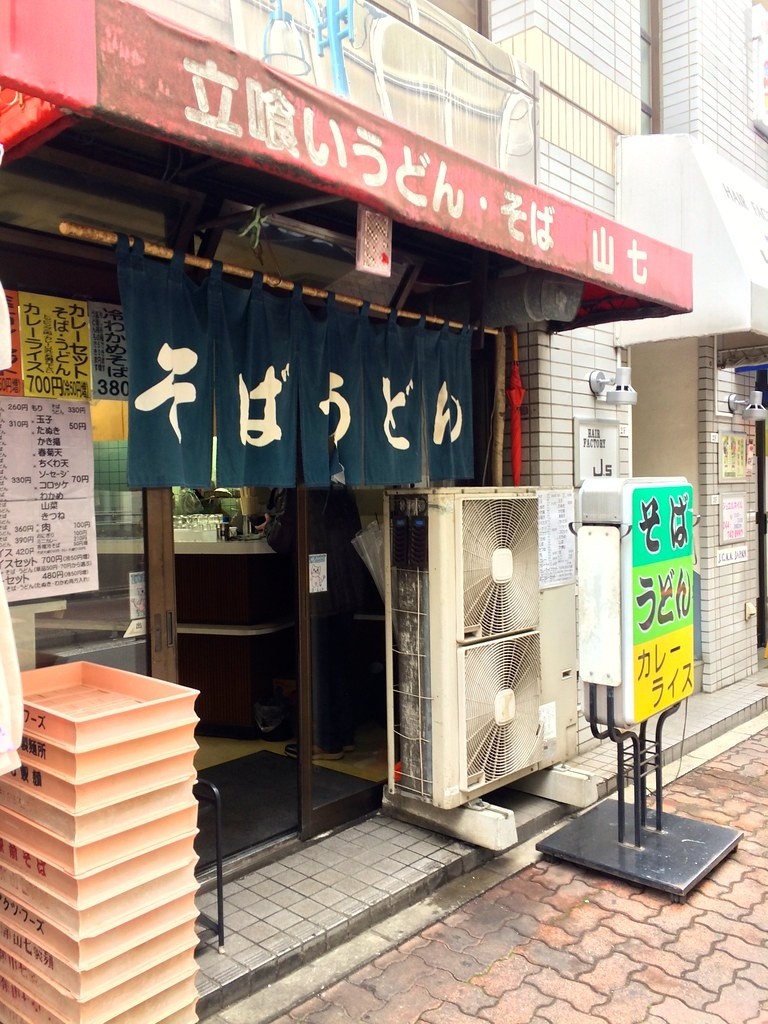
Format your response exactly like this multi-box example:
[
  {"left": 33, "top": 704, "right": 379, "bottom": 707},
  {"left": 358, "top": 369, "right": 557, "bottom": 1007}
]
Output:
[{"left": 219, "top": 516, "right": 229, "bottom": 540}]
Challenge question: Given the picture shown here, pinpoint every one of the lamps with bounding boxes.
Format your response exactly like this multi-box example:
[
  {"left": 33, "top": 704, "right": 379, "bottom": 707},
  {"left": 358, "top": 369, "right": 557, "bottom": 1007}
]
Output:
[
  {"left": 588, "top": 368, "right": 638, "bottom": 406},
  {"left": 727, "top": 390, "right": 768, "bottom": 421}
]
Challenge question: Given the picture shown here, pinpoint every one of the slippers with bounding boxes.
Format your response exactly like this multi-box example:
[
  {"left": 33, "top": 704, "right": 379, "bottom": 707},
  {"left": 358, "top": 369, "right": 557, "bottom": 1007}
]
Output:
[
  {"left": 342, "top": 741, "right": 356, "bottom": 751},
  {"left": 285, "top": 743, "right": 344, "bottom": 760}
]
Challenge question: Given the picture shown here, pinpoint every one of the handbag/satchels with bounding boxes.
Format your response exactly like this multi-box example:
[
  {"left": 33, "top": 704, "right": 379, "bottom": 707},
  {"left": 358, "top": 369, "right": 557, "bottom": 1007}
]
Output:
[{"left": 269, "top": 515, "right": 320, "bottom": 554}]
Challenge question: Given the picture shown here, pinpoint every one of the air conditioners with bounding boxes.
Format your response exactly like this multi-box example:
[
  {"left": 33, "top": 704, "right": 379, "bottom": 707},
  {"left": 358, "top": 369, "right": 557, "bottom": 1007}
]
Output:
[{"left": 383, "top": 484, "right": 578, "bottom": 812}]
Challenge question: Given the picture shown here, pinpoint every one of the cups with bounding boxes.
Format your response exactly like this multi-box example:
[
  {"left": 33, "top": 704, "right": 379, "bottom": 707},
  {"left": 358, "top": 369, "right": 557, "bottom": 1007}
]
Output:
[{"left": 174, "top": 513, "right": 221, "bottom": 527}]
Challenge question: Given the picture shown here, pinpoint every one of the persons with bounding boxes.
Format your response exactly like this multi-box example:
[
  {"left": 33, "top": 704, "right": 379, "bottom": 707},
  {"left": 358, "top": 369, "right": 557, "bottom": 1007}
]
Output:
[{"left": 252, "top": 441, "right": 363, "bottom": 762}]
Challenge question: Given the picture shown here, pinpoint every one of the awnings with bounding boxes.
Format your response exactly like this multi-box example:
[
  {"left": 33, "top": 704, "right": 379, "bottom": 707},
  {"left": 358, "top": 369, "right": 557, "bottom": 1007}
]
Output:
[{"left": 0, "top": 1, "right": 694, "bottom": 335}]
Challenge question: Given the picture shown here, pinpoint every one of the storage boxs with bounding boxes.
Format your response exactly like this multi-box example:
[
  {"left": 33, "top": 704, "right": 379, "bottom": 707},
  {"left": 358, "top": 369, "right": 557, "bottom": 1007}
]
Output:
[{"left": 0, "top": 661, "right": 203, "bottom": 1024}]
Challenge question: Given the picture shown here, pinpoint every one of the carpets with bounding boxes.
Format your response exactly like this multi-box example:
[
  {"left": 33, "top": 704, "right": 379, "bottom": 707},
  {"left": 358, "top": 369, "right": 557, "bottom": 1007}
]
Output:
[{"left": 193, "top": 749, "right": 379, "bottom": 872}]
